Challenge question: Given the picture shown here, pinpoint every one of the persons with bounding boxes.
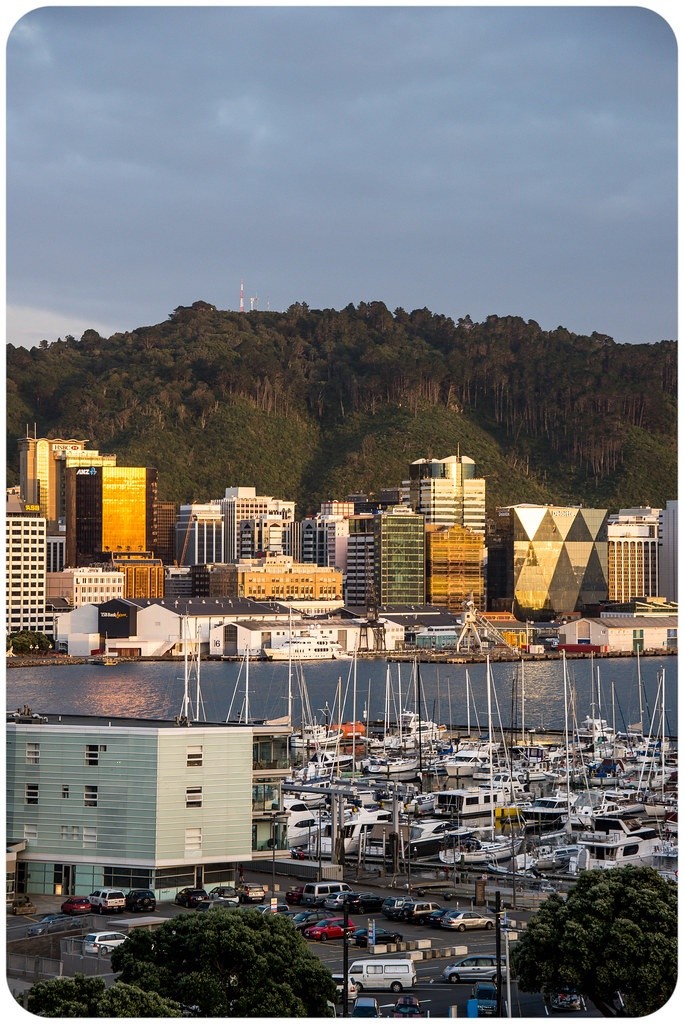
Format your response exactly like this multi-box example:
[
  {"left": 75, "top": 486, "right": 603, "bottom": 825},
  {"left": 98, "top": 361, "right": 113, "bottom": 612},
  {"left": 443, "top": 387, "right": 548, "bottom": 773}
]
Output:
[
  {"left": 238, "top": 864, "right": 245, "bottom": 884},
  {"left": 376, "top": 788, "right": 389, "bottom": 801},
  {"left": 362, "top": 766, "right": 369, "bottom": 774},
  {"left": 25, "top": 896, "right": 30, "bottom": 903}
]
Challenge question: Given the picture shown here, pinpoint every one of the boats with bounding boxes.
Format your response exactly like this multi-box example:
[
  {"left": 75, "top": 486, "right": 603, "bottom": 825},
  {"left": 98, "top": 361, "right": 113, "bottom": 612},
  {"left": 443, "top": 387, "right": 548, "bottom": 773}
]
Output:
[{"left": 173, "top": 613, "right": 679, "bottom": 892}]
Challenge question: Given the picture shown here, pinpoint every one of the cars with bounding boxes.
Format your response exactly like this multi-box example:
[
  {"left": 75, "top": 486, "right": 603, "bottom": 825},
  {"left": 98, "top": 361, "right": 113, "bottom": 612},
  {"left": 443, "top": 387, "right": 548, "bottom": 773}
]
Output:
[
  {"left": 331, "top": 974, "right": 360, "bottom": 1004},
  {"left": 27, "top": 914, "right": 89, "bottom": 938},
  {"left": 174, "top": 887, "right": 209, "bottom": 908},
  {"left": 285, "top": 886, "right": 305, "bottom": 906},
  {"left": 278, "top": 910, "right": 301, "bottom": 920},
  {"left": 291, "top": 909, "right": 338, "bottom": 936},
  {"left": 322, "top": 891, "right": 354, "bottom": 910},
  {"left": 208, "top": 886, "right": 240, "bottom": 902},
  {"left": 549, "top": 988, "right": 585, "bottom": 1010},
  {"left": 60, "top": 897, "right": 92, "bottom": 913},
  {"left": 349, "top": 927, "right": 404, "bottom": 947},
  {"left": 425, "top": 908, "right": 460, "bottom": 925},
  {"left": 82, "top": 931, "right": 131, "bottom": 956},
  {"left": 391, "top": 997, "right": 425, "bottom": 1018},
  {"left": 440, "top": 910, "right": 497, "bottom": 933},
  {"left": 305, "top": 917, "right": 356, "bottom": 941},
  {"left": 326, "top": 1000, "right": 337, "bottom": 1017}
]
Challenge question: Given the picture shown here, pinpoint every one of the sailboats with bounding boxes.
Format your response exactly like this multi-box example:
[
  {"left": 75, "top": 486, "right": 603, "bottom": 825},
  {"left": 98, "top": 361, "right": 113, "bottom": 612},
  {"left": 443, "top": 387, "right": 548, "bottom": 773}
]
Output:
[{"left": 90, "top": 631, "right": 117, "bottom": 666}]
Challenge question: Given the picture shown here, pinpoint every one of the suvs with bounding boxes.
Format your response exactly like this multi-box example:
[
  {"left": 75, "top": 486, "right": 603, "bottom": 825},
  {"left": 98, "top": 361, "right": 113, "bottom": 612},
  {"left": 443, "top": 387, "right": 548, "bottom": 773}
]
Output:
[
  {"left": 86, "top": 889, "right": 127, "bottom": 915},
  {"left": 382, "top": 896, "right": 420, "bottom": 919},
  {"left": 352, "top": 997, "right": 382, "bottom": 1017},
  {"left": 124, "top": 889, "right": 157, "bottom": 912},
  {"left": 399, "top": 901, "right": 444, "bottom": 926},
  {"left": 443, "top": 954, "right": 507, "bottom": 983},
  {"left": 237, "top": 883, "right": 268, "bottom": 902},
  {"left": 344, "top": 892, "right": 386, "bottom": 914}
]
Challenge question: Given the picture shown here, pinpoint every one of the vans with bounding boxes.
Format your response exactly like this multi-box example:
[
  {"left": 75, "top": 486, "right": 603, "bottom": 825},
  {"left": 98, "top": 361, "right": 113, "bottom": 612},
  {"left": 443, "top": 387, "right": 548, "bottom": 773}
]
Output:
[
  {"left": 473, "top": 980, "right": 498, "bottom": 1016},
  {"left": 303, "top": 881, "right": 354, "bottom": 908},
  {"left": 347, "top": 959, "right": 418, "bottom": 993},
  {"left": 253, "top": 903, "right": 290, "bottom": 916}
]
